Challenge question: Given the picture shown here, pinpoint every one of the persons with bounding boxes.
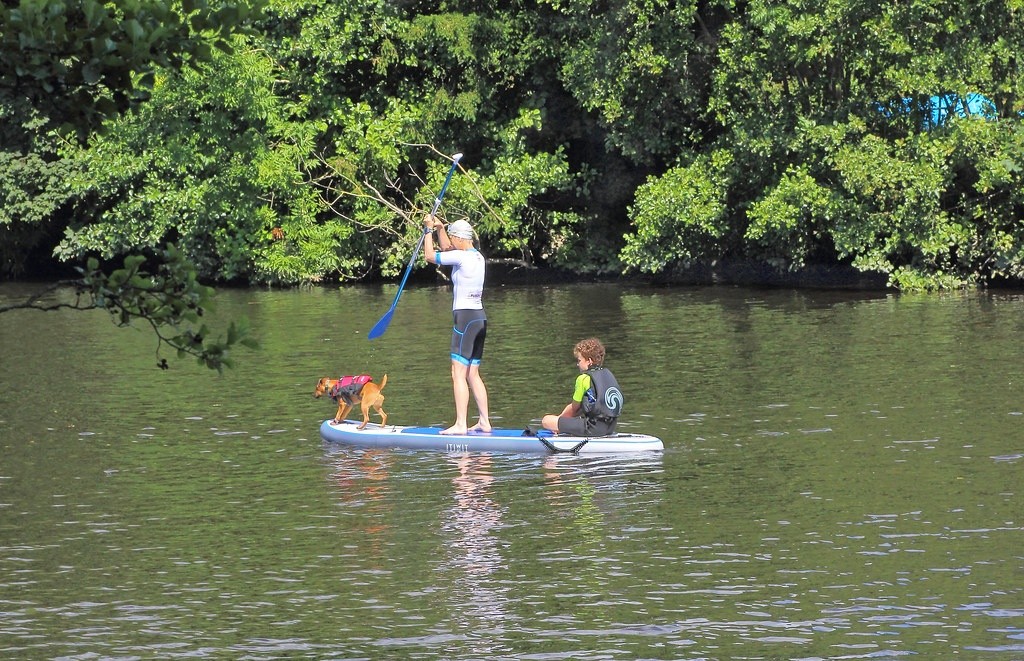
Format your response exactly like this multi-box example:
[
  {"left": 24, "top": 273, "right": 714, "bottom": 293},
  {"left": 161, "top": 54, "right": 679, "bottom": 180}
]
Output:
[
  {"left": 541, "top": 337, "right": 624, "bottom": 436},
  {"left": 423, "top": 212, "right": 492, "bottom": 433}
]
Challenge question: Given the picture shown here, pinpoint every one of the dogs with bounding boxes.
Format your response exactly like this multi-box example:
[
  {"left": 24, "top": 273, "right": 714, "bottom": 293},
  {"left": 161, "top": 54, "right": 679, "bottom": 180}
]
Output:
[{"left": 311, "top": 373, "right": 388, "bottom": 431}]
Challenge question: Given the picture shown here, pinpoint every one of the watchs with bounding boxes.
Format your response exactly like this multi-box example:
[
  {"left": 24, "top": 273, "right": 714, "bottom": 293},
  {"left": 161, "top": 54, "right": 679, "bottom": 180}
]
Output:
[{"left": 425, "top": 227, "right": 434, "bottom": 235}]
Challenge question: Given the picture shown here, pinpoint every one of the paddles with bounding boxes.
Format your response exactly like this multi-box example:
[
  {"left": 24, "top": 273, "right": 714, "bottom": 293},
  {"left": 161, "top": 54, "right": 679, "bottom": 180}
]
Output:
[{"left": 367, "top": 154, "right": 462, "bottom": 340}]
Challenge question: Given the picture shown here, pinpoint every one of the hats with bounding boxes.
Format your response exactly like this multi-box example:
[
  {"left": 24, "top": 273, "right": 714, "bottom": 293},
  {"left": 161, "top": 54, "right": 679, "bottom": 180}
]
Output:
[{"left": 447, "top": 220, "right": 473, "bottom": 239}]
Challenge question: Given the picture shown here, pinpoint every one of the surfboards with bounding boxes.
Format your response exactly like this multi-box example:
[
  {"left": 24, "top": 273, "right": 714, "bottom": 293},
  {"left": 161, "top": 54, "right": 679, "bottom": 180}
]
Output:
[{"left": 320, "top": 419, "right": 664, "bottom": 452}]
